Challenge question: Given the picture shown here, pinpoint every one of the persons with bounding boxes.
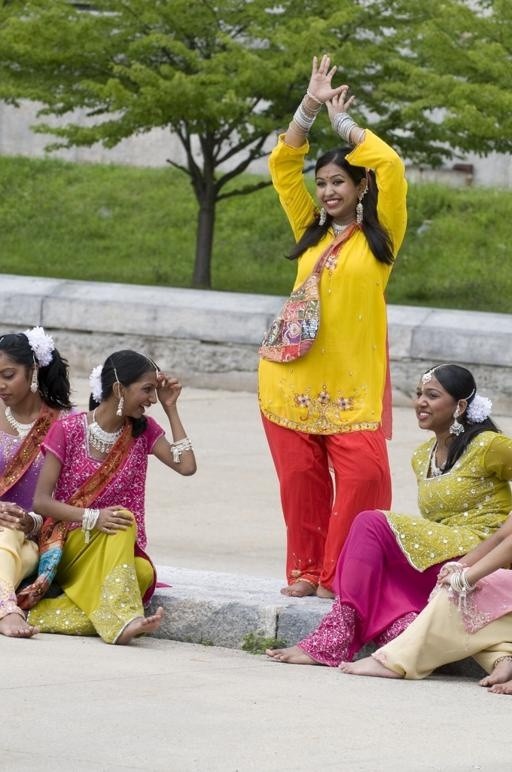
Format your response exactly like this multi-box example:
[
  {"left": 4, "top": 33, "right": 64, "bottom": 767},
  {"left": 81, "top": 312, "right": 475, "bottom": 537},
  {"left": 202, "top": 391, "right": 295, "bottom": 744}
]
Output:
[
  {"left": 256, "top": 52, "right": 410, "bottom": 598},
  {"left": 487, "top": 679, "right": 512, "bottom": 695},
  {"left": 0, "top": 323, "right": 78, "bottom": 639},
  {"left": 265, "top": 360, "right": 512, "bottom": 665},
  {"left": 336, "top": 511, "right": 512, "bottom": 686},
  {"left": 29, "top": 348, "right": 199, "bottom": 645}
]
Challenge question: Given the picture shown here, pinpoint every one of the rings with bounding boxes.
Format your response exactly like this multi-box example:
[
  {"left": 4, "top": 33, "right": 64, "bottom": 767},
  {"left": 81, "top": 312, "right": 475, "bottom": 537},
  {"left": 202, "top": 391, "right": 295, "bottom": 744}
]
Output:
[
  {"left": 112, "top": 511, "right": 118, "bottom": 517},
  {"left": 23, "top": 511, "right": 25, "bottom": 518},
  {"left": 15, "top": 517, "right": 21, "bottom": 523},
  {"left": 17, "top": 509, "right": 22, "bottom": 516}
]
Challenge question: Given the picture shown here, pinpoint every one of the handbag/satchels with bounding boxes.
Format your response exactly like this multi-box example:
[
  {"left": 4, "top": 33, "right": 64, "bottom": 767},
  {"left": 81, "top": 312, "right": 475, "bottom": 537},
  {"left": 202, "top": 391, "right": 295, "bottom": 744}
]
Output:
[
  {"left": 259, "top": 276, "right": 321, "bottom": 363},
  {"left": 17, "top": 517, "right": 70, "bottom": 609}
]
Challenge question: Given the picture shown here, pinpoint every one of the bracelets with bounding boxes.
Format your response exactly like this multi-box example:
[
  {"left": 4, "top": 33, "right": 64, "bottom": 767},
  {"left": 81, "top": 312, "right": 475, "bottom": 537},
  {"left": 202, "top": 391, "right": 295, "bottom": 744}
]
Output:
[
  {"left": 171, "top": 435, "right": 195, "bottom": 464},
  {"left": 332, "top": 111, "right": 365, "bottom": 146},
  {"left": 439, "top": 561, "right": 464, "bottom": 571},
  {"left": 289, "top": 90, "right": 325, "bottom": 136},
  {"left": 25, "top": 511, "right": 44, "bottom": 540},
  {"left": 450, "top": 566, "right": 478, "bottom": 596},
  {"left": 80, "top": 507, "right": 100, "bottom": 544}
]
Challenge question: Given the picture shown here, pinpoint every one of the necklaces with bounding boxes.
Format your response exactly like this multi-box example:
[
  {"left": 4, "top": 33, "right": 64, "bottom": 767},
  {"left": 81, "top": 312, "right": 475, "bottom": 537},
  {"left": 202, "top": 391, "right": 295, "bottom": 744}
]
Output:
[
  {"left": 330, "top": 222, "right": 351, "bottom": 234},
  {"left": 85, "top": 407, "right": 125, "bottom": 453},
  {"left": 430, "top": 443, "right": 450, "bottom": 477},
  {"left": 3, "top": 404, "right": 37, "bottom": 438}
]
